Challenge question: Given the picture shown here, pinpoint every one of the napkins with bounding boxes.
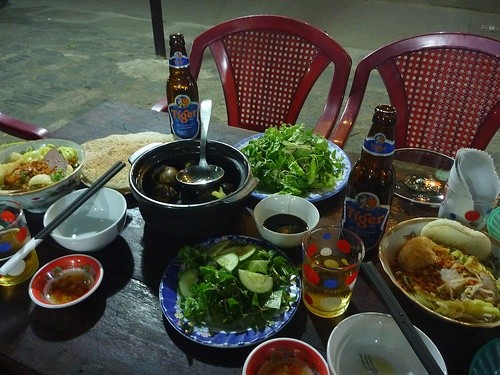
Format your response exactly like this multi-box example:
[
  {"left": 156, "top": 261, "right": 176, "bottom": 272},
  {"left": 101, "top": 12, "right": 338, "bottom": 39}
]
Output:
[{"left": 439, "top": 147, "right": 500, "bottom": 225}]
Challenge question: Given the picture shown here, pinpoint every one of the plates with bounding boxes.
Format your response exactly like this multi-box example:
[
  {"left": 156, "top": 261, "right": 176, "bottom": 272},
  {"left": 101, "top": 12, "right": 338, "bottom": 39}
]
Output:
[
  {"left": 378, "top": 217, "right": 500, "bottom": 329},
  {"left": 469, "top": 338, "right": 500, "bottom": 375},
  {"left": 231, "top": 133, "right": 353, "bottom": 202},
  {"left": 158, "top": 234, "right": 302, "bottom": 349},
  {"left": 392, "top": 147, "right": 454, "bottom": 207}
]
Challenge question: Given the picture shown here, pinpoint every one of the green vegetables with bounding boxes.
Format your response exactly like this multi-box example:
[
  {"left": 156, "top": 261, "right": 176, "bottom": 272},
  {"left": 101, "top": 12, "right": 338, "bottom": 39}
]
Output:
[
  {"left": 175, "top": 245, "right": 301, "bottom": 330},
  {"left": 20, "top": 169, "right": 66, "bottom": 183},
  {"left": 240, "top": 123, "right": 348, "bottom": 195}
]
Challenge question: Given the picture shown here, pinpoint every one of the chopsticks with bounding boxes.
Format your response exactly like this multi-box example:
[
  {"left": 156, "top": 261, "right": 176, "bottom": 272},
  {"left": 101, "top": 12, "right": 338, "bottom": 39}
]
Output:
[
  {"left": 32, "top": 160, "right": 126, "bottom": 241},
  {"left": 361, "top": 261, "right": 444, "bottom": 375}
]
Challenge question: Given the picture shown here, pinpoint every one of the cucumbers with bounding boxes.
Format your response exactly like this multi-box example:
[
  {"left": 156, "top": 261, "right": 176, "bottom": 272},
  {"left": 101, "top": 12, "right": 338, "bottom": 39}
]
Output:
[
  {"left": 179, "top": 268, "right": 197, "bottom": 299},
  {"left": 205, "top": 241, "right": 274, "bottom": 294}
]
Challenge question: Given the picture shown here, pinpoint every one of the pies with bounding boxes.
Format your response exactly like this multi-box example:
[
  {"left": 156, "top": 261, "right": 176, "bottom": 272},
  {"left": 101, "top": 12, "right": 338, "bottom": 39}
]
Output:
[{"left": 79, "top": 131, "right": 176, "bottom": 189}]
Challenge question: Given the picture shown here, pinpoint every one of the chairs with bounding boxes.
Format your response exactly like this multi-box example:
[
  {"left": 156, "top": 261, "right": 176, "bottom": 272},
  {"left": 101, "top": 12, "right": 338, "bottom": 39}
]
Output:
[
  {"left": 330, "top": 30, "right": 500, "bottom": 170},
  {"left": 150, "top": 14, "right": 352, "bottom": 139}
]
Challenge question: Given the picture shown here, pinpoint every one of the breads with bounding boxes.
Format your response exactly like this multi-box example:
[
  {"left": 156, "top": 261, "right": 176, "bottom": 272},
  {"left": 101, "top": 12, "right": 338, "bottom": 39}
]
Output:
[
  {"left": 421, "top": 219, "right": 491, "bottom": 261},
  {"left": 398, "top": 236, "right": 436, "bottom": 269}
]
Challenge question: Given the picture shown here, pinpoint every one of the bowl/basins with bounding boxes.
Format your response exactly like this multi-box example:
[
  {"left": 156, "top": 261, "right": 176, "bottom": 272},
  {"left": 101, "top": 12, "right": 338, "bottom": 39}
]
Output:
[
  {"left": 0, "top": 139, "right": 86, "bottom": 214},
  {"left": 242, "top": 338, "right": 330, "bottom": 375},
  {"left": 27, "top": 254, "right": 104, "bottom": 310},
  {"left": 254, "top": 196, "right": 320, "bottom": 249},
  {"left": 44, "top": 187, "right": 127, "bottom": 254},
  {"left": 326, "top": 313, "right": 448, "bottom": 375}
]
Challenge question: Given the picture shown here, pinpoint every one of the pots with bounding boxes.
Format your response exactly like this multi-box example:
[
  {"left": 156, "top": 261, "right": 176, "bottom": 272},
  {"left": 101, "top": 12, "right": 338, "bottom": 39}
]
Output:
[{"left": 128, "top": 137, "right": 260, "bottom": 239}]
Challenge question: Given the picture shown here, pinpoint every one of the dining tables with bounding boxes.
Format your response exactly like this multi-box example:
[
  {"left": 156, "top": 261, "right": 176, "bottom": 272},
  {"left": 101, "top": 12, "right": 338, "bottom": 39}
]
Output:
[{"left": 0, "top": 100, "right": 500, "bottom": 375}]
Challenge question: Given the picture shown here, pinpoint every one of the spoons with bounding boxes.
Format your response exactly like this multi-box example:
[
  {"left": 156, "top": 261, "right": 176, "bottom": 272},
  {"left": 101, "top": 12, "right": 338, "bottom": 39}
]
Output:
[{"left": 176, "top": 98, "right": 225, "bottom": 188}]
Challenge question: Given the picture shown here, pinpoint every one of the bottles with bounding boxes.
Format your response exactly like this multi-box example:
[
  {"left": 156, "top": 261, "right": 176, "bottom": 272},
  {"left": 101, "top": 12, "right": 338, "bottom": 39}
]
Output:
[
  {"left": 339, "top": 104, "right": 398, "bottom": 260},
  {"left": 167, "top": 33, "right": 201, "bottom": 141}
]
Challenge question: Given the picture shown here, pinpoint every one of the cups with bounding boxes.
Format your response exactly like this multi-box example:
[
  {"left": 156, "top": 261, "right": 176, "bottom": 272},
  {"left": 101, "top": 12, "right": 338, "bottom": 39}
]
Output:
[
  {"left": 436, "top": 177, "right": 500, "bottom": 230},
  {"left": 302, "top": 225, "right": 366, "bottom": 318},
  {"left": 0, "top": 200, "right": 38, "bottom": 288}
]
